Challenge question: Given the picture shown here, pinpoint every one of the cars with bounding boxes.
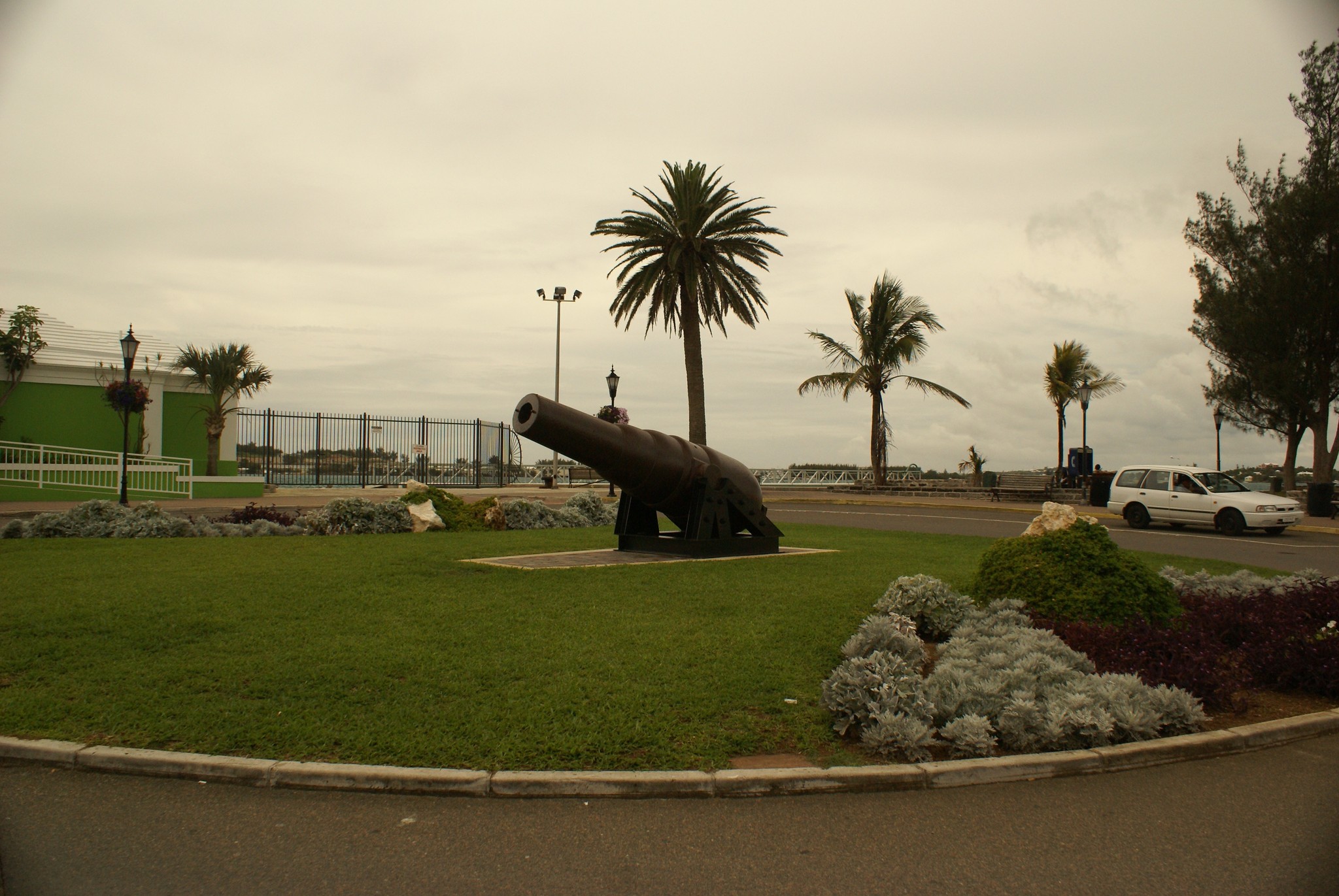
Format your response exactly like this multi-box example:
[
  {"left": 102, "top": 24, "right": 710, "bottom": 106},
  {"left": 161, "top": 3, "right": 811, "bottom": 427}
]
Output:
[{"left": 1107, "top": 465, "right": 1305, "bottom": 536}]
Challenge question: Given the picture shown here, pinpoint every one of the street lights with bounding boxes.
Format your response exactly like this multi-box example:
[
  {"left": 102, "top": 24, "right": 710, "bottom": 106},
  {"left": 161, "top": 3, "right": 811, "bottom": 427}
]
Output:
[
  {"left": 118, "top": 322, "right": 140, "bottom": 506},
  {"left": 1076, "top": 380, "right": 1094, "bottom": 506},
  {"left": 536, "top": 286, "right": 583, "bottom": 490},
  {"left": 604, "top": 364, "right": 620, "bottom": 498},
  {"left": 1213, "top": 407, "right": 1224, "bottom": 489}
]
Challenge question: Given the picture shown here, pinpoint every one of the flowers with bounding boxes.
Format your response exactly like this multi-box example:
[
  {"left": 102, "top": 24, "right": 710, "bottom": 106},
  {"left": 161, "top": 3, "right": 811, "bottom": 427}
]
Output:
[
  {"left": 592, "top": 405, "right": 630, "bottom": 424},
  {"left": 102, "top": 378, "right": 156, "bottom": 414}
]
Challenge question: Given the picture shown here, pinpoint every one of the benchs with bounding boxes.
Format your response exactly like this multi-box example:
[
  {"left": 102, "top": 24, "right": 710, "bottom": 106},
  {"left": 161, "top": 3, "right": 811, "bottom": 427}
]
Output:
[
  {"left": 990, "top": 473, "right": 1055, "bottom": 502},
  {"left": 1330, "top": 486, "right": 1339, "bottom": 520},
  {"left": 568, "top": 467, "right": 604, "bottom": 488}
]
